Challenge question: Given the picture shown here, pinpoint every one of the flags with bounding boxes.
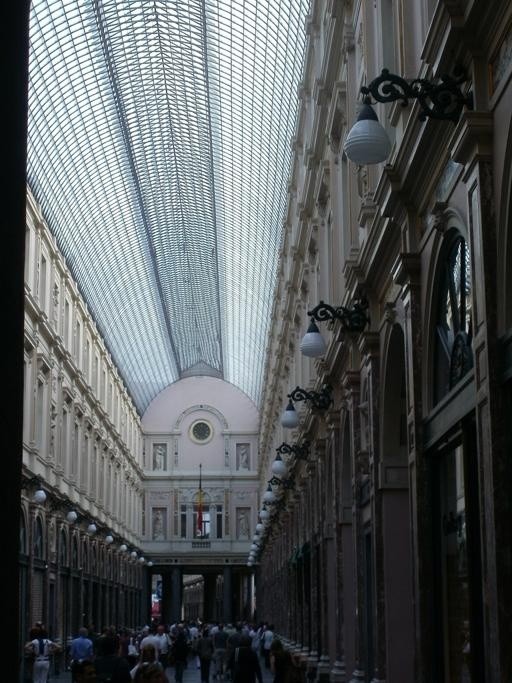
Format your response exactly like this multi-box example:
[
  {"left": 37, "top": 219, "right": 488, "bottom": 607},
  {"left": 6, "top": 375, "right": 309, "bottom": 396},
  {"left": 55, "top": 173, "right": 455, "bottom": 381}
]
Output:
[{"left": 196, "top": 473, "right": 205, "bottom": 531}]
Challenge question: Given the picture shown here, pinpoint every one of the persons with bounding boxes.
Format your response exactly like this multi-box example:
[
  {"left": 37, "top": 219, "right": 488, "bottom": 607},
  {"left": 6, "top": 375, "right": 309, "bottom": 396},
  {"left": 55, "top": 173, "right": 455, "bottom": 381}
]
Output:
[
  {"left": 238, "top": 508, "right": 247, "bottom": 530},
  {"left": 24, "top": 616, "right": 298, "bottom": 682},
  {"left": 239, "top": 444, "right": 248, "bottom": 465},
  {"left": 155, "top": 444, "right": 166, "bottom": 470},
  {"left": 155, "top": 509, "right": 164, "bottom": 533}
]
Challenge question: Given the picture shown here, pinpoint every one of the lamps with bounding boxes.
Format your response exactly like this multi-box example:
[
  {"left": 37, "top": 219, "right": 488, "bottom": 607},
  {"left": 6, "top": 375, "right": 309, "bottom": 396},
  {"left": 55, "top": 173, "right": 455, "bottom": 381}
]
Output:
[
  {"left": 245, "top": 475, "right": 297, "bottom": 567},
  {"left": 26, "top": 490, "right": 159, "bottom": 574},
  {"left": 301, "top": 296, "right": 370, "bottom": 357},
  {"left": 281, "top": 386, "right": 334, "bottom": 429},
  {"left": 342, "top": 69, "right": 471, "bottom": 166}
]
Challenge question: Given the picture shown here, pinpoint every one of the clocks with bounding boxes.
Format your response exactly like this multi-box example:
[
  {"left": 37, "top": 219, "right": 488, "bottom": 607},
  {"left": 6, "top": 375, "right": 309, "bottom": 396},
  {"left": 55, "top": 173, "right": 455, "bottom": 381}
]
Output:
[{"left": 190, "top": 421, "right": 213, "bottom": 444}]
